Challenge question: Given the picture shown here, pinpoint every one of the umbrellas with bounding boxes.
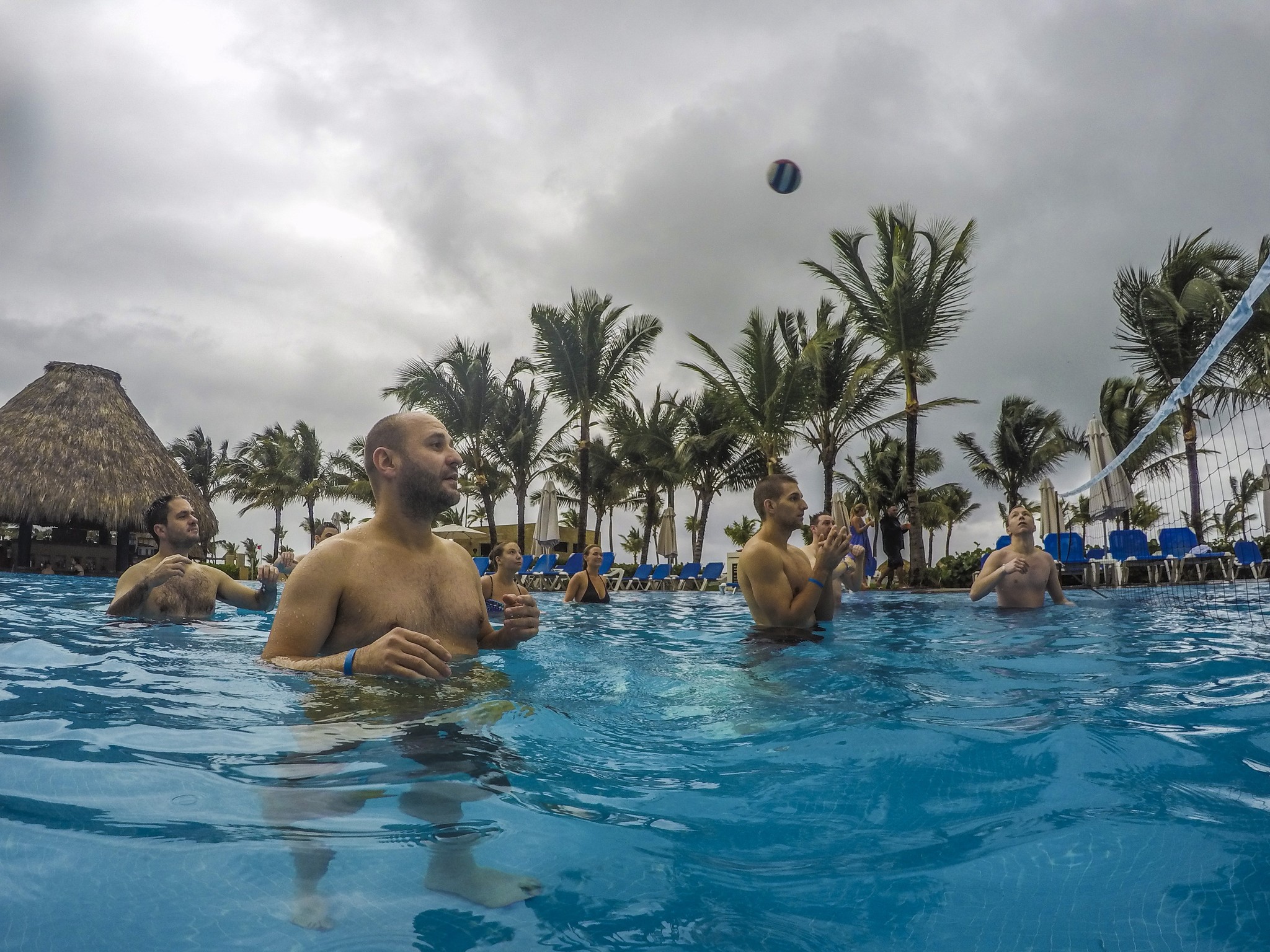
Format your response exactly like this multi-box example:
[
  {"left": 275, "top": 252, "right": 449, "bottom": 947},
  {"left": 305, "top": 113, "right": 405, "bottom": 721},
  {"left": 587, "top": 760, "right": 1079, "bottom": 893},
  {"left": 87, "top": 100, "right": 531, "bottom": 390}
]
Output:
[
  {"left": 829, "top": 490, "right": 852, "bottom": 538},
  {"left": 1085, "top": 414, "right": 1138, "bottom": 587},
  {"left": 1260, "top": 459, "right": 1269, "bottom": 535},
  {"left": 331, "top": 512, "right": 341, "bottom": 534},
  {"left": 531, "top": 479, "right": 560, "bottom": 572},
  {"left": 430, "top": 524, "right": 487, "bottom": 543},
  {"left": 657, "top": 507, "right": 678, "bottom": 578},
  {"left": 1039, "top": 478, "right": 1066, "bottom": 544}
]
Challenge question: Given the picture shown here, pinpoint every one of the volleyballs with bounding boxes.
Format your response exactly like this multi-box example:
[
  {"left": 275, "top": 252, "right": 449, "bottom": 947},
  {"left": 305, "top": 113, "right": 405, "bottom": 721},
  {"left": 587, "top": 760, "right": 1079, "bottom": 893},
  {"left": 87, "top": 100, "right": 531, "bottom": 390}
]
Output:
[{"left": 766, "top": 159, "right": 801, "bottom": 194}]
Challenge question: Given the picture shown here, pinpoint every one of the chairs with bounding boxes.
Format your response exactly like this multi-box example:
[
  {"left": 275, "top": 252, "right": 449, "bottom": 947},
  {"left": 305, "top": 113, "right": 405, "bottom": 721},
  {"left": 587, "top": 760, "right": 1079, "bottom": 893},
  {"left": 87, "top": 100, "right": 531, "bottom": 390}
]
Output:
[
  {"left": 973, "top": 526, "right": 1270, "bottom": 586},
  {"left": 468, "top": 552, "right": 738, "bottom": 595}
]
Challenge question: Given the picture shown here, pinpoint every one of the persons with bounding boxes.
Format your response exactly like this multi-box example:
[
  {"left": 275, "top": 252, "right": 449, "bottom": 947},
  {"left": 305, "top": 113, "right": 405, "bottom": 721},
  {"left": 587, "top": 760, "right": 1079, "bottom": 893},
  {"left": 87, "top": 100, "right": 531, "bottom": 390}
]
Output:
[
  {"left": 563, "top": 544, "right": 611, "bottom": 604},
  {"left": 105, "top": 494, "right": 279, "bottom": 622},
  {"left": 738, "top": 473, "right": 852, "bottom": 631},
  {"left": 970, "top": 505, "right": 1076, "bottom": 608},
  {"left": 272, "top": 522, "right": 339, "bottom": 574},
  {"left": 848, "top": 503, "right": 875, "bottom": 590},
  {"left": 875, "top": 506, "right": 910, "bottom": 590},
  {"left": 259, "top": 413, "right": 540, "bottom": 680},
  {"left": 37, "top": 556, "right": 85, "bottom": 577},
  {"left": 479, "top": 541, "right": 529, "bottom": 613},
  {"left": 470, "top": 548, "right": 480, "bottom": 557},
  {"left": 799, "top": 511, "right": 865, "bottom": 609}
]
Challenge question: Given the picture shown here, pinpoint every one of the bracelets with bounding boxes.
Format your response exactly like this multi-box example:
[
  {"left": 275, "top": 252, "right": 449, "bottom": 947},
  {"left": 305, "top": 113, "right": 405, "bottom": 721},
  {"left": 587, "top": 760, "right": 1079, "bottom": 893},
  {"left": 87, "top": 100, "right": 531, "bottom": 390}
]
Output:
[
  {"left": 343, "top": 646, "right": 358, "bottom": 676},
  {"left": 807, "top": 577, "right": 823, "bottom": 590},
  {"left": 842, "top": 561, "right": 849, "bottom": 571},
  {"left": 1002, "top": 563, "right": 1007, "bottom": 575}
]
